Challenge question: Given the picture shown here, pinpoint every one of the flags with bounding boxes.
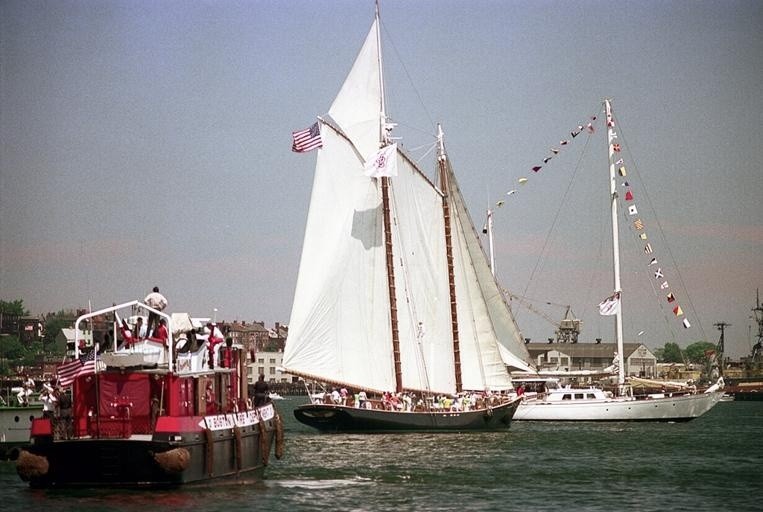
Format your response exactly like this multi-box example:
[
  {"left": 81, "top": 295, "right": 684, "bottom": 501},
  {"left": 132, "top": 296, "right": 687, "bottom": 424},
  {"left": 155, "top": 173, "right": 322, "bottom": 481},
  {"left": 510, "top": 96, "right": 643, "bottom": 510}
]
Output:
[
  {"left": 292, "top": 122, "right": 322, "bottom": 152},
  {"left": 56, "top": 348, "right": 96, "bottom": 386},
  {"left": 365, "top": 143, "right": 398, "bottom": 177},
  {"left": 487, "top": 116, "right": 596, "bottom": 217},
  {"left": 608, "top": 118, "right": 692, "bottom": 328},
  {"left": 598, "top": 292, "right": 620, "bottom": 316}
]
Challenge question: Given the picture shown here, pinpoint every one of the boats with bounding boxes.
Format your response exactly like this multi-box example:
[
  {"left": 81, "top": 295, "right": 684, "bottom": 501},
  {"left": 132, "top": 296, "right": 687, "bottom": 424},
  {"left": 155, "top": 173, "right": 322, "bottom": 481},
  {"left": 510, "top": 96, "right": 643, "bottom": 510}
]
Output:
[{"left": 16, "top": 294, "right": 288, "bottom": 489}]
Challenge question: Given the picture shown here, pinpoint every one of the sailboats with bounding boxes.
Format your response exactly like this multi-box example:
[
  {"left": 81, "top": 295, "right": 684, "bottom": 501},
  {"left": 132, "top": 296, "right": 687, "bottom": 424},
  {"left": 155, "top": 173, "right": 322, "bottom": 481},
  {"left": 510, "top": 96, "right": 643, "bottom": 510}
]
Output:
[
  {"left": 715, "top": 288, "right": 763, "bottom": 402},
  {"left": 274, "top": 0, "right": 524, "bottom": 437},
  {"left": 474, "top": 93, "right": 727, "bottom": 423}
]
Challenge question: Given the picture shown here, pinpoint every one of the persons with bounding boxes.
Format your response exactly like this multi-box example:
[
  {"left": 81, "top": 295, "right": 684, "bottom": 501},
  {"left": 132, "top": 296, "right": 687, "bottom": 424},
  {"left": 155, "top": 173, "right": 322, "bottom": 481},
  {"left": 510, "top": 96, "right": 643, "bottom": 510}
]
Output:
[
  {"left": 380, "top": 391, "right": 417, "bottom": 412},
  {"left": 205, "top": 322, "right": 225, "bottom": 368},
  {"left": 254, "top": 374, "right": 268, "bottom": 406},
  {"left": 16, "top": 374, "right": 35, "bottom": 407},
  {"left": 433, "top": 392, "right": 478, "bottom": 412},
  {"left": 38, "top": 378, "right": 71, "bottom": 418},
  {"left": 327, "top": 385, "right": 354, "bottom": 406},
  {"left": 517, "top": 385, "right": 526, "bottom": 402},
  {"left": 249, "top": 333, "right": 256, "bottom": 363},
  {"left": 358, "top": 389, "right": 367, "bottom": 408},
  {"left": 131, "top": 287, "right": 168, "bottom": 340}
]
